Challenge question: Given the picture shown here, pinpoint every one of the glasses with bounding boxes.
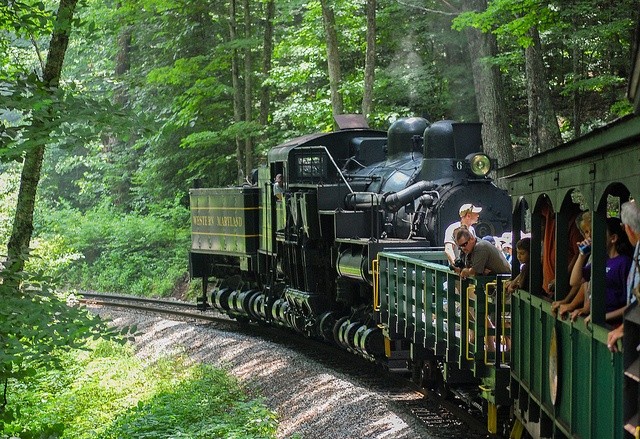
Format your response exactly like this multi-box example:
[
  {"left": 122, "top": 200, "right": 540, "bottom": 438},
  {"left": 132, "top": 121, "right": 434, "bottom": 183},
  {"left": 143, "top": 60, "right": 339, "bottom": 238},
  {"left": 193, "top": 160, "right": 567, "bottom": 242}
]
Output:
[{"left": 458, "top": 239, "right": 471, "bottom": 250}]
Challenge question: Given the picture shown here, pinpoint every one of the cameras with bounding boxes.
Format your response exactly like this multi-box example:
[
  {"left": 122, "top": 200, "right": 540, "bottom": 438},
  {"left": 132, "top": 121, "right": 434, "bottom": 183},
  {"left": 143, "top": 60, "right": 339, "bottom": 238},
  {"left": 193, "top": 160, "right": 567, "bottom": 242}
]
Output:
[{"left": 450, "top": 260, "right": 470, "bottom": 273}]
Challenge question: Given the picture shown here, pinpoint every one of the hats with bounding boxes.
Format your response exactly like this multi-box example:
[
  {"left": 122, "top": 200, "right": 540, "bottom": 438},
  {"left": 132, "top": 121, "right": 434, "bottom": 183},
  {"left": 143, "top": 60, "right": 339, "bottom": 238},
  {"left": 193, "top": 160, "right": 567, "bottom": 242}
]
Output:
[{"left": 458, "top": 204, "right": 483, "bottom": 217}]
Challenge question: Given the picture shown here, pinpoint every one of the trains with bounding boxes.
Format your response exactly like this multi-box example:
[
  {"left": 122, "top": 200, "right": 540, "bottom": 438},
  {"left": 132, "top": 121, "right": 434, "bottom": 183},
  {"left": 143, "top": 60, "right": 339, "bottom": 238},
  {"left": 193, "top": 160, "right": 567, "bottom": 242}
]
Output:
[{"left": 188, "top": 114, "right": 640, "bottom": 439}]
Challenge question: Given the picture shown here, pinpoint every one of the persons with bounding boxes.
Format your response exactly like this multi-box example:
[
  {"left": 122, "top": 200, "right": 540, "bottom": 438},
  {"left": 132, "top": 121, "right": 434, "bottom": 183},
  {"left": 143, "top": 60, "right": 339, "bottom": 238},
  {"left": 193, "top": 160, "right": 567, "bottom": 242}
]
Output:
[
  {"left": 273, "top": 173, "right": 283, "bottom": 200},
  {"left": 569, "top": 216, "right": 635, "bottom": 330},
  {"left": 444, "top": 204, "right": 483, "bottom": 293},
  {"left": 620, "top": 199, "right": 640, "bottom": 352},
  {"left": 605, "top": 321, "right": 624, "bottom": 354},
  {"left": 452, "top": 228, "right": 483, "bottom": 344},
  {"left": 482, "top": 230, "right": 531, "bottom": 264},
  {"left": 504, "top": 237, "right": 530, "bottom": 293},
  {"left": 558, "top": 210, "right": 592, "bottom": 323},
  {"left": 550, "top": 211, "right": 586, "bottom": 317},
  {"left": 456, "top": 229, "right": 513, "bottom": 354}
]
